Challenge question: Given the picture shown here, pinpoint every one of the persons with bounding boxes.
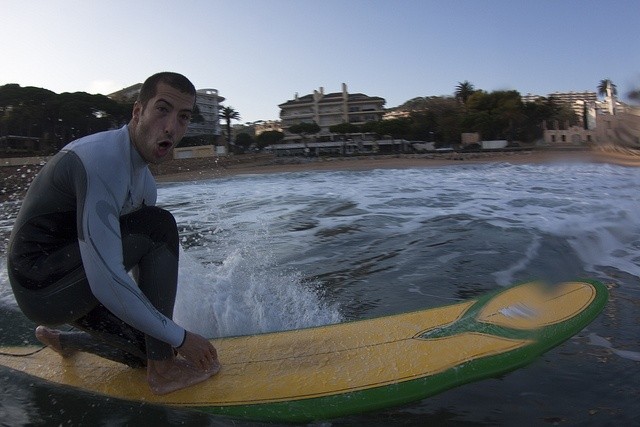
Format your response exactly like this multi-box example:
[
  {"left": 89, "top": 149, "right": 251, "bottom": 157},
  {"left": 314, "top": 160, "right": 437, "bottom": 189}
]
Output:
[{"left": 7, "top": 71, "right": 222, "bottom": 396}]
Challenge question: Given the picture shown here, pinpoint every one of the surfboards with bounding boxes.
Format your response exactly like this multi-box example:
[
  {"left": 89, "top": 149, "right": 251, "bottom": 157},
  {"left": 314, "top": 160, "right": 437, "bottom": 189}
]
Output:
[{"left": 0, "top": 275, "right": 605, "bottom": 419}]
